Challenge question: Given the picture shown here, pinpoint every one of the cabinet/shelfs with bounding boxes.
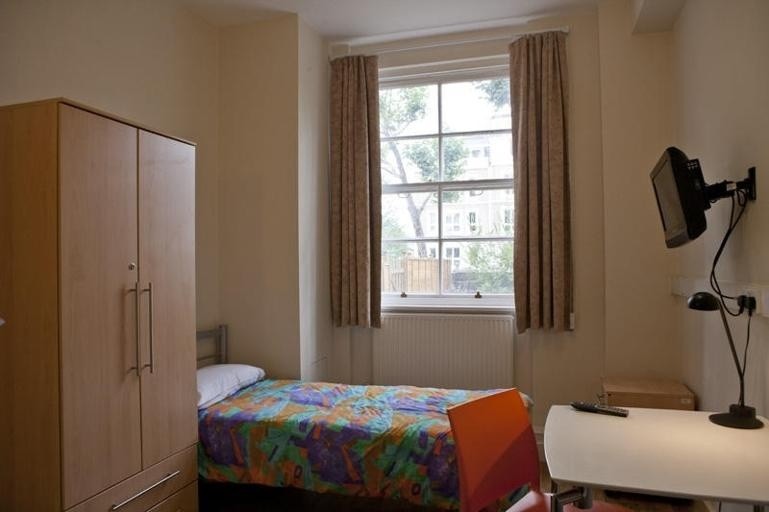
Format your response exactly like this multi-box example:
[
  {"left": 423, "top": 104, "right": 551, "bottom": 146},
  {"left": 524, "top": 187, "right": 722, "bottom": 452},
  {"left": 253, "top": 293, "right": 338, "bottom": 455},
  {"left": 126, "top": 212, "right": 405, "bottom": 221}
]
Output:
[
  {"left": 0, "top": 96, "right": 196, "bottom": 512},
  {"left": 597, "top": 375, "right": 697, "bottom": 505}
]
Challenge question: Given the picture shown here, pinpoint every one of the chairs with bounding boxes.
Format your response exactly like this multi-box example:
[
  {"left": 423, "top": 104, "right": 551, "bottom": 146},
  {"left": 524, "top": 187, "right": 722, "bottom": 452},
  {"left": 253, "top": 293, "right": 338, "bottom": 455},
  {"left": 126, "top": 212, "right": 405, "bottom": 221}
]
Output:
[{"left": 447, "top": 385, "right": 636, "bottom": 512}]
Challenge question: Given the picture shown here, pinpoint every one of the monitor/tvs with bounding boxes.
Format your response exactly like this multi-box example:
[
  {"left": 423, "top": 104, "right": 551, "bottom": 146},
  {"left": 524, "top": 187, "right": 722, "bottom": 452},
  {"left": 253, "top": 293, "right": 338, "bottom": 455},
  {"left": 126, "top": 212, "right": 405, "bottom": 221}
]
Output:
[{"left": 648, "top": 145, "right": 712, "bottom": 250}]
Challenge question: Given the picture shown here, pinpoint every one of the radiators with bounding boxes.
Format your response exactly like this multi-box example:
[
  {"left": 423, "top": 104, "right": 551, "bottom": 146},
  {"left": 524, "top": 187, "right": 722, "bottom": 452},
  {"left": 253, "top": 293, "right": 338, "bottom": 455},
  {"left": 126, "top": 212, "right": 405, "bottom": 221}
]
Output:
[{"left": 371, "top": 311, "right": 516, "bottom": 391}]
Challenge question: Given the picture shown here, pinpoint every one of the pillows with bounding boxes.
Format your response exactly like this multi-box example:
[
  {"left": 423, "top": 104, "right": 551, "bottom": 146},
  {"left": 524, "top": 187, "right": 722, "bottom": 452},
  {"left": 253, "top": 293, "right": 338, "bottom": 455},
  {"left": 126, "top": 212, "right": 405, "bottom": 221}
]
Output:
[{"left": 196, "top": 362, "right": 265, "bottom": 411}]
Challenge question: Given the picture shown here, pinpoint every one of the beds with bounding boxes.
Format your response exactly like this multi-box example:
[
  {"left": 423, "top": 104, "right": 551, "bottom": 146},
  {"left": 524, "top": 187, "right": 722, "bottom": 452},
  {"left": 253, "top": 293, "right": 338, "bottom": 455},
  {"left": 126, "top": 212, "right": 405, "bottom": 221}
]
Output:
[{"left": 194, "top": 322, "right": 534, "bottom": 512}]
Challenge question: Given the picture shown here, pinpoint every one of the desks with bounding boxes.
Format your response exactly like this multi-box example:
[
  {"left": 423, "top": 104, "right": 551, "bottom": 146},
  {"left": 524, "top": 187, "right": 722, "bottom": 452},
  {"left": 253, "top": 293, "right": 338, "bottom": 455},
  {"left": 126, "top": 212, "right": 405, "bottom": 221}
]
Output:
[{"left": 544, "top": 402, "right": 769, "bottom": 512}]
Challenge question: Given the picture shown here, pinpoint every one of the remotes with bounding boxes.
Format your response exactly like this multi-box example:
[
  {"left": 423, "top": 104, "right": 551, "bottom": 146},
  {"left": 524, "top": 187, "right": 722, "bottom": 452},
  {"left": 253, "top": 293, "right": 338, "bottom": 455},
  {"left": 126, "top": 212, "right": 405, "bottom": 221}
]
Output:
[{"left": 569, "top": 398, "right": 629, "bottom": 418}]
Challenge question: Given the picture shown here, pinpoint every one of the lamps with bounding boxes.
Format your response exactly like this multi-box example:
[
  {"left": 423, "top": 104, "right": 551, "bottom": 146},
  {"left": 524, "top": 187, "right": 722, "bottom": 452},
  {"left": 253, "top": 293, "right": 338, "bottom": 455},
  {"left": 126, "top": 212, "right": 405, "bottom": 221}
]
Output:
[{"left": 686, "top": 291, "right": 764, "bottom": 430}]
